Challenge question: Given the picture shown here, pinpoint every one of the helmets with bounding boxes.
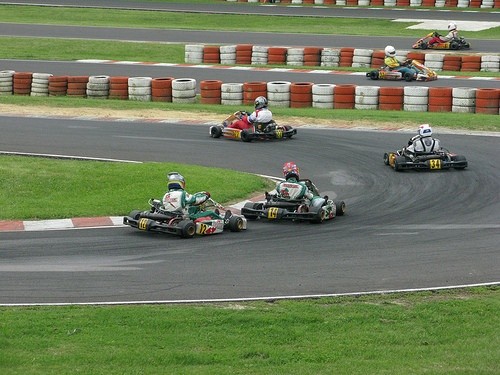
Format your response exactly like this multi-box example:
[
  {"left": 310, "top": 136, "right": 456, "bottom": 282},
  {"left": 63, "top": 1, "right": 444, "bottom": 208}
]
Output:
[
  {"left": 384, "top": 45, "right": 396, "bottom": 58},
  {"left": 281, "top": 161, "right": 300, "bottom": 177},
  {"left": 166, "top": 171, "right": 185, "bottom": 190},
  {"left": 447, "top": 23, "right": 457, "bottom": 31},
  {"left": 254, "top": 95, "right": 269, "bottom": 108},
  {"left": 417, "top": 123, "right": 433, "bottom": 137}
]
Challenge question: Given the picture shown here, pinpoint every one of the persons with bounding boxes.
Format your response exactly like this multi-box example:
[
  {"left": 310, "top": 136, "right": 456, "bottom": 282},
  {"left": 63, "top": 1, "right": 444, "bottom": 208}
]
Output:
[
  {"left": 384, "top": 45, "right": 423, "bottom": 74},
  {"left": 419, "top": 23, "right": 460, "bottom": 47},
  {"left": 403, "top": 124, "right": 443, "bottom": 156},
  {"left": 275, "top": 162, "right": 328, "bottom": 201},
  {"left": 222, "top": 96, "right": 273, "bottom": 130},
  {"left": 162, "top": 172, "right": 221, "bottom": 219}
]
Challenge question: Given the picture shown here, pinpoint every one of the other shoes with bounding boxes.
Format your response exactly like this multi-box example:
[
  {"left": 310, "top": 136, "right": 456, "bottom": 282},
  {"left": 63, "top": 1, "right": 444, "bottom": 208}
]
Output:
[
  {"left": 223, "top": 121, "right": 227, "bottom": 127},
  {"left": 323, "top": 195, "right": 328, "bottom": 204},
  {"left": 421, "top": 43, "right": 427, "bottom": 49},
  {"left": 224, "top": 209, "right": 232, "bottom": 222}
]
